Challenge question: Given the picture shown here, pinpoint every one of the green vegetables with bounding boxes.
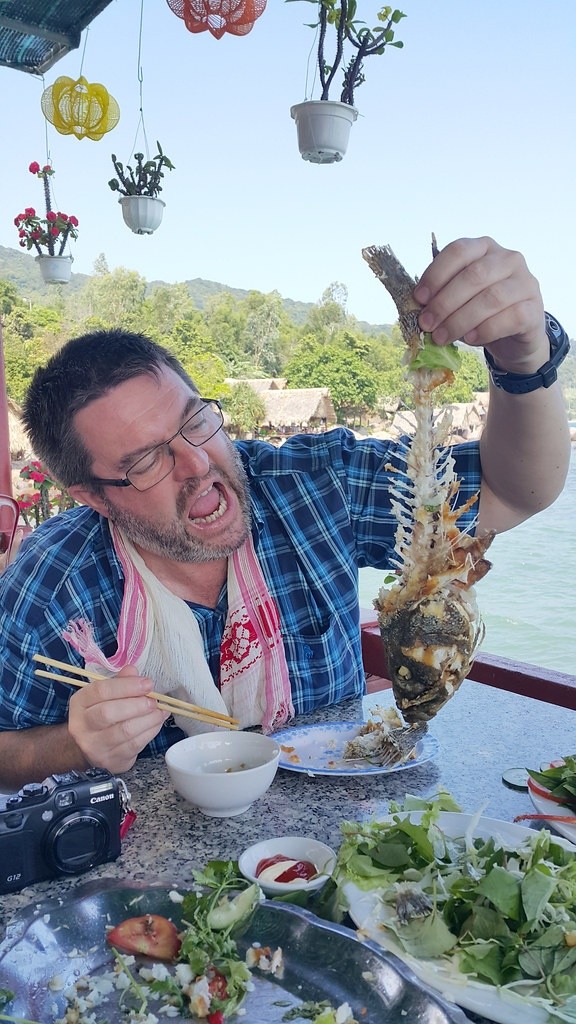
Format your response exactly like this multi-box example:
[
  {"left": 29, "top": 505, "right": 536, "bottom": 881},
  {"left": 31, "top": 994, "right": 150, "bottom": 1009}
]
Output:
[{"left": 111, "top": 754, "right": 576, "bottom": 1024}]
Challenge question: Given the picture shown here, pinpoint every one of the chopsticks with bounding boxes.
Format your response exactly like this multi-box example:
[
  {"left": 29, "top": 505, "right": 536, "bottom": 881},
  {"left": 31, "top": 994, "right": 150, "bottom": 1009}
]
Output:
[{"left": 32, "top": 654, "right": 240, "bottom": 731}]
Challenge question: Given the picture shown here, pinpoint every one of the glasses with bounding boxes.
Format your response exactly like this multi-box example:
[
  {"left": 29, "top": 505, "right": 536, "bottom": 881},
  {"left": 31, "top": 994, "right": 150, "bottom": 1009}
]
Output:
[{"left": 68, "top": 398, "right": 224, "bottom": 493}]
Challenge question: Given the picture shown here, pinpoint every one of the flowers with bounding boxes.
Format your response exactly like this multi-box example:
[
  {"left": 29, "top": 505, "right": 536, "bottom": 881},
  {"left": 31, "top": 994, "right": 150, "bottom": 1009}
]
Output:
[
  {"left": 14, "top": 162, "right": 78, "bottom": 255},
  {"left": 15, "top": 461, "right": 79, "bottom": 530}
]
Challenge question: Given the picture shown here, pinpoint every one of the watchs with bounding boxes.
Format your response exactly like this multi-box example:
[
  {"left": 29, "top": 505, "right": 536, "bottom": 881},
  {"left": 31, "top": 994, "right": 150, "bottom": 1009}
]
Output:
[{"left": 483, "top": 312, "right": 570, "bottom": 394}]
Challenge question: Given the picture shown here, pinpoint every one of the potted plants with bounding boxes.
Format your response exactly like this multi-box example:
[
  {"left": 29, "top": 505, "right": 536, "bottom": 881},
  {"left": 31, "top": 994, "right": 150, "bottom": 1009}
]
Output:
[
  {"left": 107, "top": 139, "right": 175, "bottom": 234},
  {"left": 288, "top": 0, "right": 408, "bottom": 166}
]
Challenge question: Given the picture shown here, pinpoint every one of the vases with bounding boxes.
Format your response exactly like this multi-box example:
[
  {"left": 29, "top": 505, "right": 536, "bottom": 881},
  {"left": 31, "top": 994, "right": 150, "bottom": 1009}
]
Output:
[{"left": 34, "top": 253, "right": 75, "bottom": 284}]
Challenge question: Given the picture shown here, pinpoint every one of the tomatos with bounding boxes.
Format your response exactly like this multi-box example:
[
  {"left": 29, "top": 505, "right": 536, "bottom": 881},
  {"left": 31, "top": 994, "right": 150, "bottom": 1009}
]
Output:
[{"left": 104, "top": 915, "right": 180, "bottom": 960}]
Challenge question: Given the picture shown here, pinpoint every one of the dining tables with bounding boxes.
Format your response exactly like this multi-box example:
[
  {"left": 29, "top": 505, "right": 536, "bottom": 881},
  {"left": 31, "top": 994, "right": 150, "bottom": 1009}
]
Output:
[{"left": 0, "top": 678, "right": 575, "bottom": 1024}]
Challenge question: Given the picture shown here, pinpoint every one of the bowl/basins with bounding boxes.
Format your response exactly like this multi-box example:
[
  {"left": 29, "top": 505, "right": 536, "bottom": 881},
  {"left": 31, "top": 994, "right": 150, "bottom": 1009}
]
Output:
[
  {"left": 238, "top": 837, "right": 338, "bottom": 895},
  {"left": 165, "top": 731, "right": 281, "bottom": 817}
]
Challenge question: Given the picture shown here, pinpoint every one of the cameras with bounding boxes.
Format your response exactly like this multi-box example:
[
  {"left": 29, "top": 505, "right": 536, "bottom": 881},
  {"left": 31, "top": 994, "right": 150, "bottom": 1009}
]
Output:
[{"left": 0, "top": 767, "right": 122, "bottom": 893}]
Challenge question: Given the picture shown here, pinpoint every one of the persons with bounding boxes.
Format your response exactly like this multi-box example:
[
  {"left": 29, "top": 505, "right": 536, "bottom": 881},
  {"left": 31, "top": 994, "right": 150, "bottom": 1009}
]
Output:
[{"left": 0, "top": 236, "right": 571, "bottom": 793}]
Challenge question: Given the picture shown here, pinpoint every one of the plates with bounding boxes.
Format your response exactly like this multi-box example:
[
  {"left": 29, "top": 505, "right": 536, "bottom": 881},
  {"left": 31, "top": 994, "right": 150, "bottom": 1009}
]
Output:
[
  {"left": 0, "top": 879, "right": 474, "bottom": 1023},
  {"left": 339, "top": 811, "right": 576, "bottom": 1023},
  {"left": 528, "top": 778, "right": 576, "bottom": 843},
  {"left": 266, "top": 722, "right": 440, "bottom": 775}
]
